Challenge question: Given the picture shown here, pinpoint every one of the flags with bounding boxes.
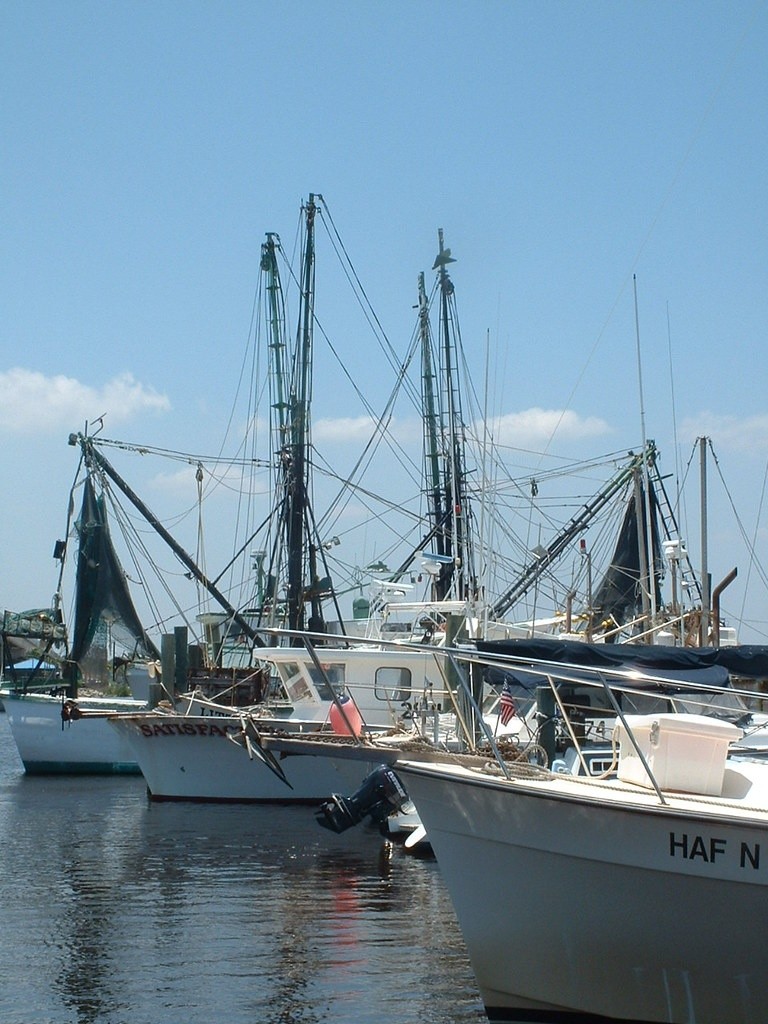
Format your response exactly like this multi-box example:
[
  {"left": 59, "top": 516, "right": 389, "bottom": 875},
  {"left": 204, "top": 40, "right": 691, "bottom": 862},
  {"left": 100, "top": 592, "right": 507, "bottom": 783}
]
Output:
[{"left": 500, "top": 677, "right": 516, "bottom": 727}]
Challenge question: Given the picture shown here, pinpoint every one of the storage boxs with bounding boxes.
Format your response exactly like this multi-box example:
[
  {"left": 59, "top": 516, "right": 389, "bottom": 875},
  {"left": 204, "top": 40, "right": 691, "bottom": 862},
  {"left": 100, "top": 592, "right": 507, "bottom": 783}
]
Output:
[{"left": 615, "top": 713, "right": 744, "bottom": 796}]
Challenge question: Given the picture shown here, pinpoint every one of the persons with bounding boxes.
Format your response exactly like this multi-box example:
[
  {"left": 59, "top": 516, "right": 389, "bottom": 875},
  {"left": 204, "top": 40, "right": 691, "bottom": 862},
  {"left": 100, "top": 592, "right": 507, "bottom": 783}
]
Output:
[
  {"left": 262, "top": 606, "right": 270, "bottom": 612},
  {"left": 236, "top": 635, "right": 246, "bottom": 643}
]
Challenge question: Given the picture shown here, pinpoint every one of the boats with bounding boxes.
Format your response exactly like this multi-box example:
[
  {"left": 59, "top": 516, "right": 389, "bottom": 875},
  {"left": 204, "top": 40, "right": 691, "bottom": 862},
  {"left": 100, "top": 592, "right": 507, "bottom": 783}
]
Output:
[{"left": 225, "top": 639, "right": 768, "bottom": 1024}]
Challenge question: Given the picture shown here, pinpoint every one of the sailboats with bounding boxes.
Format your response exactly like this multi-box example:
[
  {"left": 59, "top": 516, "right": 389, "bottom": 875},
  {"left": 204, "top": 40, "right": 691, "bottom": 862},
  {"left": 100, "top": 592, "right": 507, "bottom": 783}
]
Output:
[{"left": 0, "top": 193, "right": 767, "bottom": 805}]
